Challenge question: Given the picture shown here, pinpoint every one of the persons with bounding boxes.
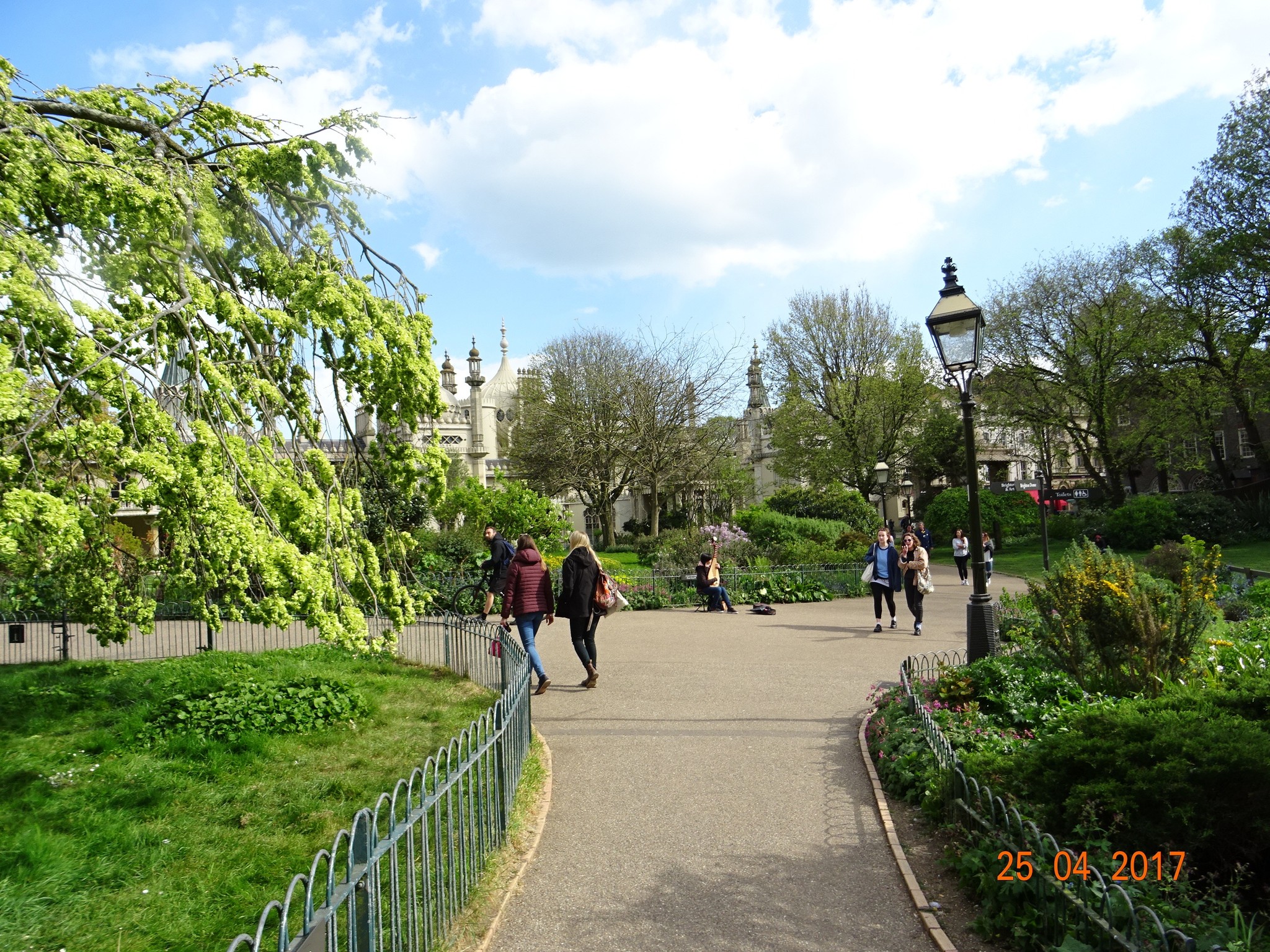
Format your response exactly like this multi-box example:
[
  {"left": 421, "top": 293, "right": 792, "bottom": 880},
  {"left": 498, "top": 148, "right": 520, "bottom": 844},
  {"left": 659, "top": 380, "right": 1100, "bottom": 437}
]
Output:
[
  {"left": 501, "top": 533, "right": 555, "bottom": 695},
  {"left": 864, "top": 513, "right": 993, "bottom": 636},
  {"left": 467, "top": 525, "right": 518, "bottom": 626},
  {"left": 696, "top": 553, "right": 739, "bottom": 614},
  {"left": 557, "top": 530, "right": 607, "bottom": 688}
]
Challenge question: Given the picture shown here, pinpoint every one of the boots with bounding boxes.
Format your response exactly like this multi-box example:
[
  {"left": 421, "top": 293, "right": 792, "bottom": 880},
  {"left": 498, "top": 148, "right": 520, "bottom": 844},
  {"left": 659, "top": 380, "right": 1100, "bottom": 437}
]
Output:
[
  {"left": 586, "top": 660, "right": 599, "bottom": 689},
  {"left": 581, "top": 678, "right": 588, "bottom": 686}
]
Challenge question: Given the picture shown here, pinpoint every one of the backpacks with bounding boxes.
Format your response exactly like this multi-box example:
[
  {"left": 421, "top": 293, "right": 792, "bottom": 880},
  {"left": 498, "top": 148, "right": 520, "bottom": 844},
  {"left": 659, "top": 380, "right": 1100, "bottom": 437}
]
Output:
[
  {"left": 592, "top": 561, "right": 618, "bottom": 609},
  {"left": 495, "top": 539, "right": 516, "bottom": 566}
]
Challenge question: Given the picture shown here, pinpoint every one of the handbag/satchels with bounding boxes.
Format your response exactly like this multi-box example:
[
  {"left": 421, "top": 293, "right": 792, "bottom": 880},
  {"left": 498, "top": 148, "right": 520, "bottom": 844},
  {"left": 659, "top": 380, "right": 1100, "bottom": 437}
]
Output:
[
  {"left": 917, "top": 547, "right": 934, "bottom": 594},
  {"left": 604, "top": 590, "right": 628, "bottom": 619},
  {"left": 861, "top": 542, "right": 876, "bottom": 583},
  {"left": 489, "top": 624, "right": 504, "bottom": 658},
  {"left": 963, "top": 537, "right": 971, "bottom": 559},
  {"left": 750, "top": 605, "right": 776, "bottom": 615}
]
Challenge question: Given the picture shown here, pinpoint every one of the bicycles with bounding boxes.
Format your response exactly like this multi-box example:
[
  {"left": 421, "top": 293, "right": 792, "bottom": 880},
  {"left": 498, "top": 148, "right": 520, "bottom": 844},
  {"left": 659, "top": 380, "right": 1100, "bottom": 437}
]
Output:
[{"left": 452, "top": 561, "right": 548, "bottom": 624}]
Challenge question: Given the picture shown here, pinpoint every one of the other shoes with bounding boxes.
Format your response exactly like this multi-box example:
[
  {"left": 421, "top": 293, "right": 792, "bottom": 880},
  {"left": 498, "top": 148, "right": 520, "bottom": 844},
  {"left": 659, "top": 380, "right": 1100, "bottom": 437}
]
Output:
[
  {"left": 988, "top": 578, "right": 991, "bottom": 584},
  {"left": 535, "top": 675, "right": 551, "bottom": 694},
  {"left": 965, "top": 580, "right": 969, "bottom": 585},
  {"left": 874, "top": 624, "right": 882, "bottom": 632},
  {"left": 986, "top": 582, "right": 989, "bottom": 587},
  {"left": 510, "top": 619, "right": 517, "bottom": 625},
  {"left": 961, "top": 581, "right": 964, "bottom": 585},
  {"left": 710, "top": 608, "right": 724, "bottom": 613},
  {"left": 891, "top": 620, "right": 897, "bottom": 628},
  {"left": 914, "top": 621, "right": 917, "bottom": 629},
  {"left": 472, "top": 614, "right": 486, "bottom": 625},
  {"left": 727, "top": 608, "right": 739, "bottom": 613},
  {"left": 914, "top": 628, "right": 921, "bottom": 635}
]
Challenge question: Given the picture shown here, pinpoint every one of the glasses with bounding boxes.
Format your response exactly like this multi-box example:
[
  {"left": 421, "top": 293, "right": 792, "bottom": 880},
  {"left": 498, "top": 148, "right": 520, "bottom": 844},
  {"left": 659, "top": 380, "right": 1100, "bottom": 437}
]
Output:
[
  {"left": 907, "top": 527, "right": 912, "bottom": 529},
  {"left": 903, "top": 539, "right": 913, "bottom": 542}
]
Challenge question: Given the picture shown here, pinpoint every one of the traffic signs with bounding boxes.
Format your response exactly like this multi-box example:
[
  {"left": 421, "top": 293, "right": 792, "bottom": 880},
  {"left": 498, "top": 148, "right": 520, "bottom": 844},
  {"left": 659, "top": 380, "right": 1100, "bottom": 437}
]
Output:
[
  {"left": 990, "top": 481, "right": 1017, "bottom": 495},
  {"left": 1014, "top": 479, "right": 1041, "bottom": 492}
]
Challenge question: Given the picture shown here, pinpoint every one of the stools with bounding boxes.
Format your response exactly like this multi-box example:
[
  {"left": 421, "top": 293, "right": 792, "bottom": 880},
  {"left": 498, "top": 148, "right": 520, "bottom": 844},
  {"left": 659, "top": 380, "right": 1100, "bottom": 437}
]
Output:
[{"left": 694, "top": 591, "right": 712, "bottom": 612}]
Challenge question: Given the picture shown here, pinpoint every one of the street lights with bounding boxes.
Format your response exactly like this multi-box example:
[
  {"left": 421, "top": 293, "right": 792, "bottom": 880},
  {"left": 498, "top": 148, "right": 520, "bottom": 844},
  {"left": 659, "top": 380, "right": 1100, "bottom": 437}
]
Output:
[
  {"left": 900, "top": 472, "right": 913, "bottom": 525},
  {"left": 873, "top": 450, "right": 890, "bottom": 528},
  {"left": 926, "top": 255, "right": 1004, "bottom": 667}
]
